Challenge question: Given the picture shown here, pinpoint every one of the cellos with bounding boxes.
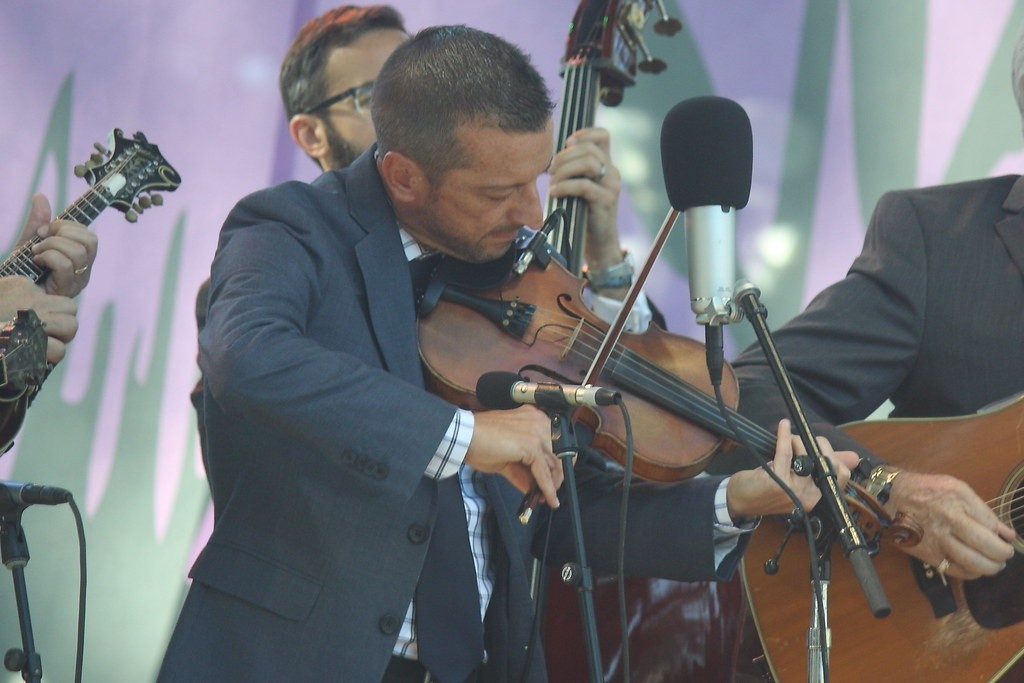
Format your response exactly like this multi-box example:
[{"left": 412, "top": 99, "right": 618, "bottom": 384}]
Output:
[{"left": 522, "top": 0, "right": 762, "bottom": 683}]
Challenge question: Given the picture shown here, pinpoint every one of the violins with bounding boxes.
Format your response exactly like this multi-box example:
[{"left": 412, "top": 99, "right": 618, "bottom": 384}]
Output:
[{"left": 415, "top": 228, "right": 923, "bottom": 550}]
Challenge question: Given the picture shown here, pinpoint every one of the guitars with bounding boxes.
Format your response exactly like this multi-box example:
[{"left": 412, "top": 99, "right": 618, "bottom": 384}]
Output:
[
  {"left": 1, "top": 127, "right": 183, "bottom": 459},
  {"left": 718, "top": 392, "right": 1023, "bottom": 683}
]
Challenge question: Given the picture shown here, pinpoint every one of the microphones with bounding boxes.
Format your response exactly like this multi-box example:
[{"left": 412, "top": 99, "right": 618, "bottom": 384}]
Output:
[
  {"left": 0, "top": 481, "right": 70, "bottom": 506},
  {"left": 659, "top": 95, "right": 754, "bottom": 385},
  {"left": 475, "top": 370, "right": 621, "bottom": 407}
]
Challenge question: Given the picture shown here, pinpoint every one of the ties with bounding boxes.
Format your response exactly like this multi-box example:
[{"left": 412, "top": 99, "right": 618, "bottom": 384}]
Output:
[{"left": 416, "top": 363, "right": 486, "bottom": 683}]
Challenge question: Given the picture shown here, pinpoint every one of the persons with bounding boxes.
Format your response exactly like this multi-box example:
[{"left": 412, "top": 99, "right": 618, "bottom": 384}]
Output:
[
  {"left": 155, "top": 5, "right": 858, "bottom": 683},
  {"left": 722, "top": 41, "right": 1024, "bottom": 683},
  {"left": 0, "top": 191, "right": 98, "bottom": 366}
]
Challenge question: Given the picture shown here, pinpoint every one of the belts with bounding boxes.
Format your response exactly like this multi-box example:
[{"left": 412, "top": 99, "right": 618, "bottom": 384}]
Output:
[{"left": 382, "top": 655, "right": 438, "bottom": 682}]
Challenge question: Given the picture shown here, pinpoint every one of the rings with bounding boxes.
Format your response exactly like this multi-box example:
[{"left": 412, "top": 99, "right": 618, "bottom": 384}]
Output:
[
  {"left": 936, "top": 558, "right": 951, "bottom": 575},
  {"left": 592, "top": 164, "right": 607, "bottom": 183},
  {"left": 75, "top": 265, "right": 87, "bottom": 276}
]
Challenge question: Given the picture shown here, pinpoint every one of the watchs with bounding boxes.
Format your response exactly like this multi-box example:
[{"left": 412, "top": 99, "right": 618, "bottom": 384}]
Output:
[{"left": 867, "top": 465, "right": 901, "bottom": 497}]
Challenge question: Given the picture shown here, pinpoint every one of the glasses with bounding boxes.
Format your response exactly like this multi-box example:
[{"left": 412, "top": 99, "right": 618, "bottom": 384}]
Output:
[{"left": 305, "top": 82, "right": 373, "bottom": 116}]
